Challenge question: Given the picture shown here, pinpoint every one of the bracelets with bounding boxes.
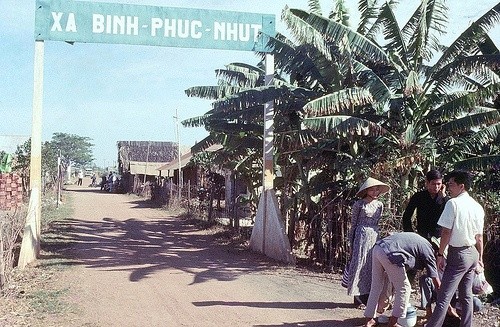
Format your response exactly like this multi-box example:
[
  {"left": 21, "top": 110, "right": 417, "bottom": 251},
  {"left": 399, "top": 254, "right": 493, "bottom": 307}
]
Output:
[{"left": 436, "top": 252, "right": 443, "bottom": 257}]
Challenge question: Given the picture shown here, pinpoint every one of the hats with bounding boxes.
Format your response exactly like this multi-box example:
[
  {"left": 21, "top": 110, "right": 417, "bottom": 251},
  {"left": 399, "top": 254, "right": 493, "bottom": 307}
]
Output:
[
  {"left": 431, "top": 236, "right": 449, "bottom": 259},
  {"left": 356, "top": 177, "right": 391, "bottom": 198}
]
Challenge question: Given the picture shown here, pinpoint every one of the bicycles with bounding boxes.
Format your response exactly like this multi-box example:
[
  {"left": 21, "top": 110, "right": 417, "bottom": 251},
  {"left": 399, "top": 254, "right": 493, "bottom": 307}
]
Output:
[{"left": 92, "top": 179, "right": 97, "bottom": 188}]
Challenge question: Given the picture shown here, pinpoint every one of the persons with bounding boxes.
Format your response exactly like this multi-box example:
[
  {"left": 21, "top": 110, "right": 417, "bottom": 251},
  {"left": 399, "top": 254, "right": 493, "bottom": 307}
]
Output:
[
  {"left": 100, "top": 170, "right": 114, "bottom": 193},
  {"left": 77, "top": 170, "right": 83, "bottom": 185},
  {"left": 402, "top": 169, "right": 451, "bottom": 242},
  {"left": 376, "top": 262, "right": 418, "bottom": 313},
  {"left": 419, "top": 259, "right": 482, "bottom": 326},
  {"left": 362, "top": 232, "right": 461, "bottom": 327},
  {"left": 91, "top": 174, "right": 96, "bottom": 186},
  {"left": 426, "top": 170, "right": 486, "bottom": 327},
  {"left": 342, "top": 176, "right": 391, "bottom": 310}
]
